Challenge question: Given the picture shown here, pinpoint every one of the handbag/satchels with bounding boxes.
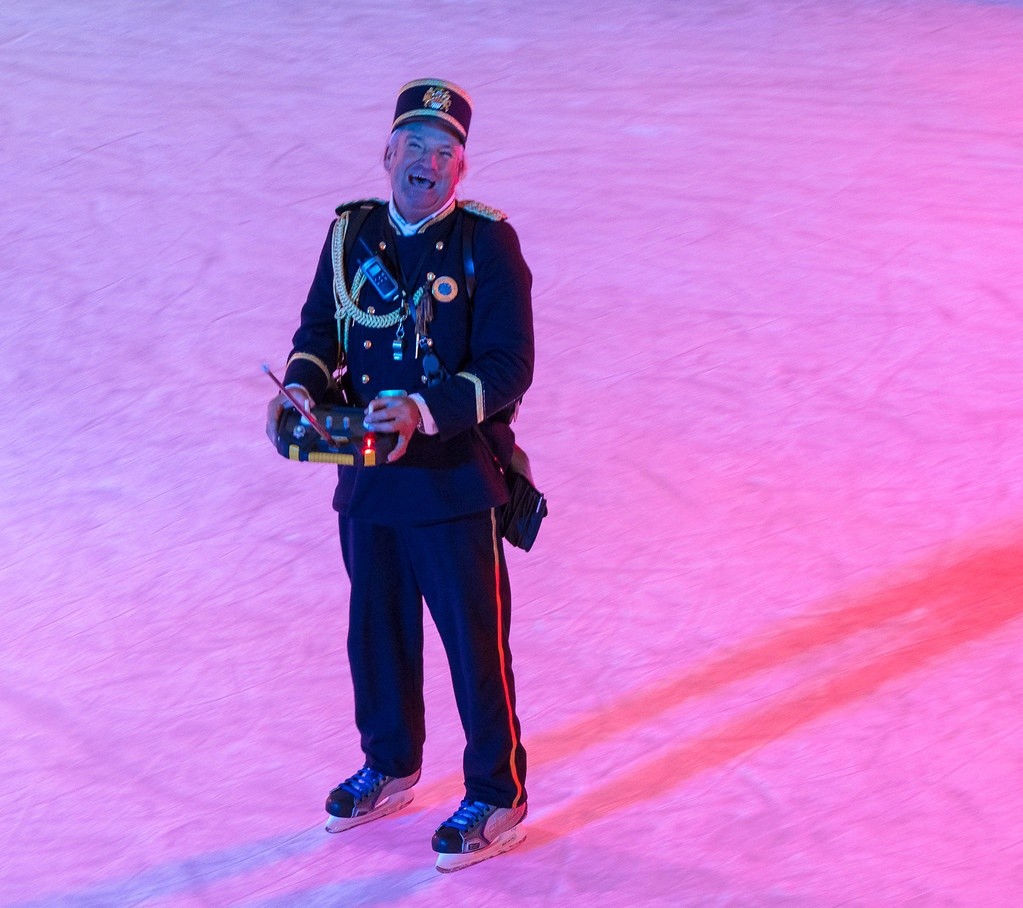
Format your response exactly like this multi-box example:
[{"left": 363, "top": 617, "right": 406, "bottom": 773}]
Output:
[{"left": 499, "top": 444, "right": 547, "bottom": 553}]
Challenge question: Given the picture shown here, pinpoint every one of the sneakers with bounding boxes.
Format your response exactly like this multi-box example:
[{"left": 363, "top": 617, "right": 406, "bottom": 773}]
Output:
[
  {"left": 432, "top": 798, "right": 528, "bottom": 873},
  {"left": 325, "top": 765, "right": 421, "bottom": 833}
]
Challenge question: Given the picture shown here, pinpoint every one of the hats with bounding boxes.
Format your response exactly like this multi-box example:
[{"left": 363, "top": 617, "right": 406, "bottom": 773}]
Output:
[{"left": 391, "top": 78, "right": 474, "bottom": 149}]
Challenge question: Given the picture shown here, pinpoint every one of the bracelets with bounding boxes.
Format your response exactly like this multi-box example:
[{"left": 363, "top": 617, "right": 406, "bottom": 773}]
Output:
[{"left": 417, "top": 414, "right": 424, "bottom": 430}]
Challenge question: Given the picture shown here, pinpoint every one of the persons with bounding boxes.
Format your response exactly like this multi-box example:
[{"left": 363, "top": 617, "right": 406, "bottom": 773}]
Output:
[{"left": 266, "top": 78, "right": 535, "bottom": 854}]
